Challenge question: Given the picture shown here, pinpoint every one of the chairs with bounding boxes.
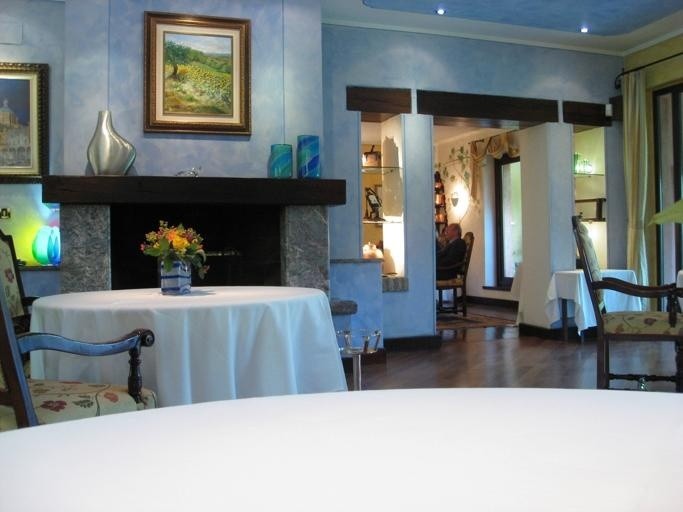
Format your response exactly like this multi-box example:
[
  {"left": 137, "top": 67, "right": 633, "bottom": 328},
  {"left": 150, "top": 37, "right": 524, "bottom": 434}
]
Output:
[
  {"left": 435, "top": 233, "right": 474, "bottom": 319},
  {"left": 0, "top": 275, "right": 158, "bottom": 433},
  {"left": 0, "top": 228, "right": 43, "bottom": 392},
  {"left": 573, "top": 217, "right": 683, "bottom": 394}
]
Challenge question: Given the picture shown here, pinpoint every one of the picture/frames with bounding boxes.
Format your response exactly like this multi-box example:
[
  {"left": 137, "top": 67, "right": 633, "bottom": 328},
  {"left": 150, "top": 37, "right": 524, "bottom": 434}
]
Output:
[
  {"left": 142, "top": 10, "right": 252, "bottom": 137},
  {"left": 0, "top": 61, "right": 51, "bottom": 184}
]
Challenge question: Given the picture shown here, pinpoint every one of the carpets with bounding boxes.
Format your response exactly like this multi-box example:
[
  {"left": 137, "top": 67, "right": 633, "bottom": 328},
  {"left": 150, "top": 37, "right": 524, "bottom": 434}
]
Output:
[{"left": 436, "top": 309, "right": 518, "bottom": 330}]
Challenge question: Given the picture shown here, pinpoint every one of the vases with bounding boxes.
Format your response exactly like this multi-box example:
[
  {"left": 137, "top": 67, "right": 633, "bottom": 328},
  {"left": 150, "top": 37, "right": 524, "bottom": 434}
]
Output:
[
  {"left": 267, "top": 143, "right": 292, "bottom": 178},
  {"left": 160, "top": 259, "right": 191, "bottom": 295},
  {"left": 298, "top": 136, "right": 321, "bottom": 177}
]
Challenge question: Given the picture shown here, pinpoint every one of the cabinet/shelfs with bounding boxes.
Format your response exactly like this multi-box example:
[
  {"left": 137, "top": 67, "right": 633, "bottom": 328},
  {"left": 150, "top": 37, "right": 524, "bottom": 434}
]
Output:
[{"left": 360, "top": 110, "right": 403, "bottom": 278}]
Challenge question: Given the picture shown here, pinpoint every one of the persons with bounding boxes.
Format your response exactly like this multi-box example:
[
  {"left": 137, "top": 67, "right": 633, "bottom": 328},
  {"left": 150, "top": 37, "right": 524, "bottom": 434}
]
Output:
[{"left": 436, "top": 223, "right": 467, "bottom": 280}]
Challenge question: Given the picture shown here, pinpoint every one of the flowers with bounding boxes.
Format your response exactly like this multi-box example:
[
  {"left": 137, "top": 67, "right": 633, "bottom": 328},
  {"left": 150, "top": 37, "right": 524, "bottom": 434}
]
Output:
[{"left": 141, "top": 221, "right": 208, "bottom": 279}]
